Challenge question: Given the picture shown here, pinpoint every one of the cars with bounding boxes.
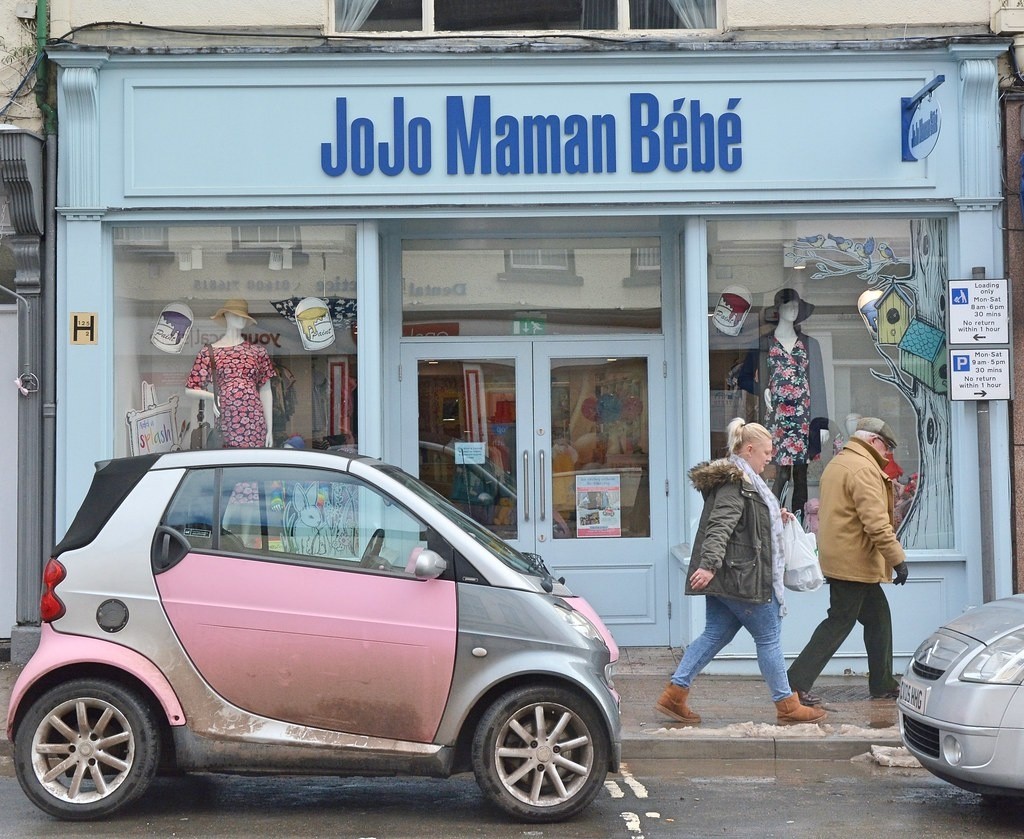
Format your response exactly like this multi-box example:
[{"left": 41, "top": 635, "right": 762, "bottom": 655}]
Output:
[
  {"left": 6, "top": 447, "right": 624, "bottom": 824},
  {"left": 418, "top": 442, "right": 571, "bottom": 537},
  {"left": 896, "top": 592, "right": 1024, "bottom": 796}
]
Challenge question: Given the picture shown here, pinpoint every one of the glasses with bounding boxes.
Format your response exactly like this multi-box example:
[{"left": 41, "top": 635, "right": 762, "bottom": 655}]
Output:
[{"left": 877, "top": 436, "right": 894, "bottom": 454}]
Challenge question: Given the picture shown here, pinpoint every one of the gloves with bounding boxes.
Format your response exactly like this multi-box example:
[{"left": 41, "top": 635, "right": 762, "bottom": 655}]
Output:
[{"left": 893, "top": 562, "right": 908, "bottom": 585}]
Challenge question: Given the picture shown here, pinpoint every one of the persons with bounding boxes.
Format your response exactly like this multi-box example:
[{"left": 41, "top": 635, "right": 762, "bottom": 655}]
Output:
[
  {"left": 654, "top": 416, "right": 830, "bottom": 725},
  {"left": 786, "top": 416, "right": 909, "bottom": 707},
  {"left": 185, "top": 297, "right": 277, "bottom": 546},
  {"left": 737, "top": 288, "right": 830, "bottom": 522}
]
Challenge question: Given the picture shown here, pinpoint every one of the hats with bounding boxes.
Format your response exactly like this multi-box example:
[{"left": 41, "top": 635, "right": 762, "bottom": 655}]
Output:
[
  {"left": 856, "top": 417, "right": 897, "bottom": 448},
  {"left": 765, "top": 289, "right": 813, "bottom": 324},
  {"left": 209, "top": 299, "right": 256, "bottom": 332}
]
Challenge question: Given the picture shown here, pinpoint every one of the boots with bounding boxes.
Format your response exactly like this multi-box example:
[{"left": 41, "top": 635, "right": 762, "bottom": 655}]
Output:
[
  {"left": 654, "top": 683, "right": 702, "bottom": 724},
  {"left": 774, "top": 691, "right": 828, "bottom": 728}
]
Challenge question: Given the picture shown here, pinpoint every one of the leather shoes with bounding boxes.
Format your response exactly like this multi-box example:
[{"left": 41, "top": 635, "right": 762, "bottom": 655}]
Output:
[
  {"left": 888, "top": 686, "right": 901, "bottom": 697},
  {"left": 796, "top": 690, "right": 821, "bottom": 704}
]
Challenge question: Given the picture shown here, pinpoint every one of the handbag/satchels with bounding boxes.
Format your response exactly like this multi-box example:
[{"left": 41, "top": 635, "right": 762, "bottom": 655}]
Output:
[
  {"left": 190, "top": 421, "right": 222, "bottom": 450},
  {"left": 781, "top": 514, "right": 826, "bottom": 593}
]
